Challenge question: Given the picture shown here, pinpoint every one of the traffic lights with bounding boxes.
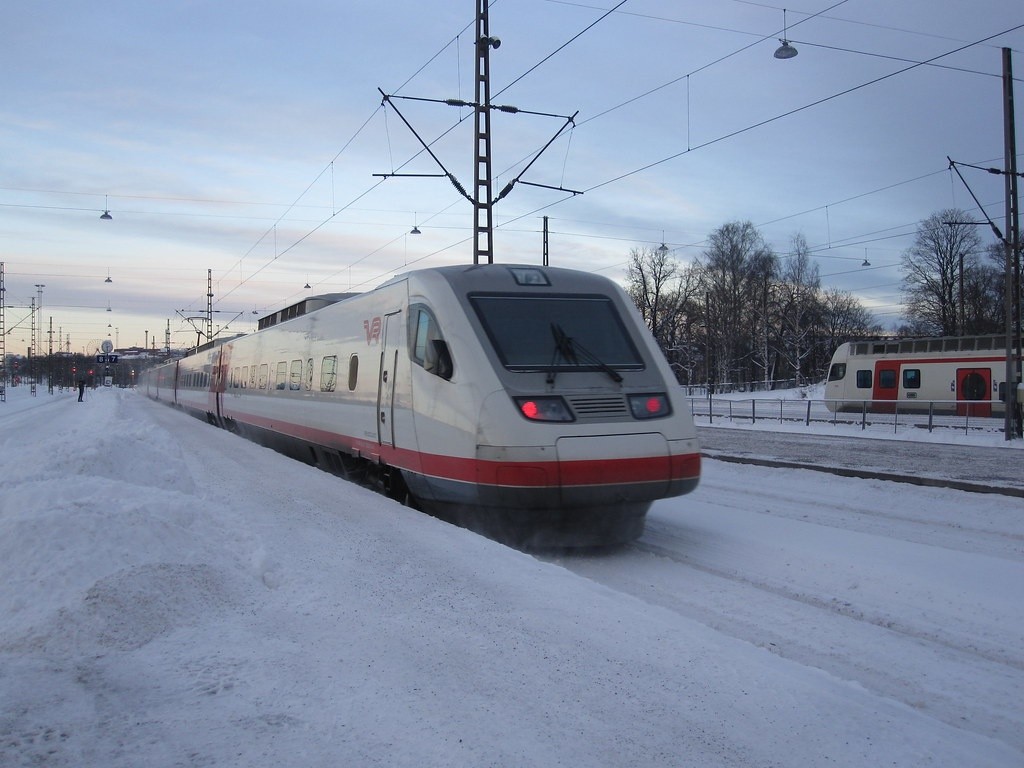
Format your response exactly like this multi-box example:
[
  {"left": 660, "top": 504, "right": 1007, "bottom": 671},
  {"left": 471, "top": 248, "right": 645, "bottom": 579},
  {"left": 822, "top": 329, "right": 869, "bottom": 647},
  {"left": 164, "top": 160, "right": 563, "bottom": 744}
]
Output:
[
  {"left": 15, "top": 364, "right": 18, "bottom": 368},
  {"left": 72, "top": 367, "right": 76, "bottom": 372},
  {"left": 131, "top": 369, "right": 134, "bottom": 376},
  {"left": 89, "top": 370, "right": 94, "bottom": 375},
  {"left": 83, "top": 370, "right": 85, "bottom": 374}
]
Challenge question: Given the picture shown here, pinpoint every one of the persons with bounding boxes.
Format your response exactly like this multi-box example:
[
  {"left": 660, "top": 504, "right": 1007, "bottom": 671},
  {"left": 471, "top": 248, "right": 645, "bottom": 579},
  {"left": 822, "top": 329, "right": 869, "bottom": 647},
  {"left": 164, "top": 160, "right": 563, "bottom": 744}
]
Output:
[{"left": 77, "top": 377, "right": 84, "bottom": 402}]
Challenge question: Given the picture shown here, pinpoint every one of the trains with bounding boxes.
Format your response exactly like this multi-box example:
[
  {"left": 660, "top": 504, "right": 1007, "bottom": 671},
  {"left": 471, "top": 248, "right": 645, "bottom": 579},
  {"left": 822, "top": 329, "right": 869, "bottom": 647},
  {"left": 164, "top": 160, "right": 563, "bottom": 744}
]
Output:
[
  {"left": 137, "top": 264, "right": 700, "bottom": 546},
  {"left": 824, "top": 334, "right": 1023, "bottom": 418}
]
